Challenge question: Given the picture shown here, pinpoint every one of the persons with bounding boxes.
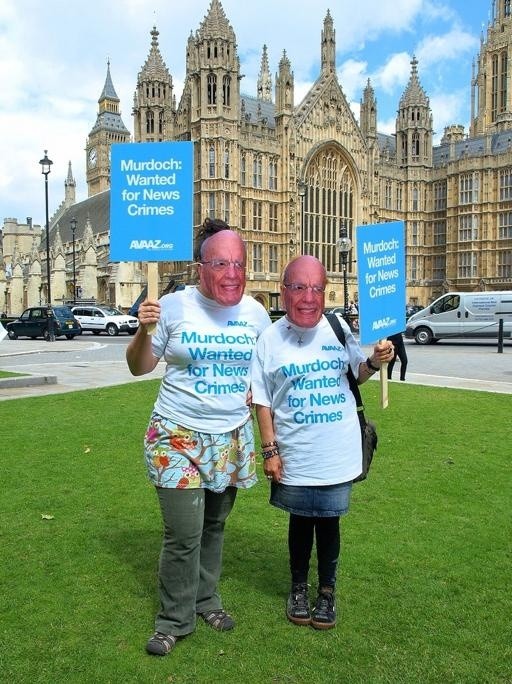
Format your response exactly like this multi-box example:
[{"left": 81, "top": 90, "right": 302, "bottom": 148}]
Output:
[
  {"left": 281, "top": 255, "right": 326, "bottom": 327},
  {"left": 198, "top": 230, "right": 246, "bottom": 305},
  {"left": 251, "top": 255, "right": 394, "bottom": 627},
  {"left": 127, "top": 219, "right": 275, "bottom": 655},
  {"left": 387, "top": 332, "right": 408, "bottom": 381},
  {"left": 349, "top": 300, "right": 358, "bottom": 313}
]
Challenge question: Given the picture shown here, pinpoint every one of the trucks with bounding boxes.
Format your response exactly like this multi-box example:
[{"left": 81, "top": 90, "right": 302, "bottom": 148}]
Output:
[
  {"left": 127, "top": 281, "right": 287, "bottom": 326},
  {"left": 406, "top": 290, "right": 512, "bottom": 346}
]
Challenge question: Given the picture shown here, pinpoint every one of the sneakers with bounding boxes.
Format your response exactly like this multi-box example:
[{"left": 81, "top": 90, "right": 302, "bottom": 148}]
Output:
[
  {"left": 286, "top": 582, "right": 313, "bottom": 626},
  {"left": 311, "top": 582, "right": 338, "bottom": 630},
  {"left": 145, "top": 631, "right": 178, "bottom": 657},
  {"left": 200, "top": 608, "right": 235, "bottom": 632}
]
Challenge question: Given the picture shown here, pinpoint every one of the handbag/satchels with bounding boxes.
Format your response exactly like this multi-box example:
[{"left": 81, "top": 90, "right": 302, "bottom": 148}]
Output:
[{"left": 353, "top": 410, "right": 378, "bottom": 484}]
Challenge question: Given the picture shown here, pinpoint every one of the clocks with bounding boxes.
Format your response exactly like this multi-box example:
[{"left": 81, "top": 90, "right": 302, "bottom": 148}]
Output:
[{"left": 88, "top": 148, "right": 97, "bottom": 169}]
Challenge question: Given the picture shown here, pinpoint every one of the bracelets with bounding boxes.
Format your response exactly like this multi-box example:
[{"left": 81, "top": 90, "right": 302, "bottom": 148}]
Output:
[
  {"left": 261, "top": 448, "right": 279, "bottom": 458},
  {"left": 366, "top": 357, "right": 380, "bottom": 371},
  {"left": 261, "top": 441, "right": 277, "bottom": 448}
]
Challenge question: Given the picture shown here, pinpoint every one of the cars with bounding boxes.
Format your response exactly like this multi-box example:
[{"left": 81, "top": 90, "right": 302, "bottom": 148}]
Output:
[{"left": 325, "top": 307, "right": 344, "bottom": 317}]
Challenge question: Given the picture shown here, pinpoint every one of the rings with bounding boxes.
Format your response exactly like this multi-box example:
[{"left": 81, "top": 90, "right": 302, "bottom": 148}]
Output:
[
  {"left": 389, "top": 347, "right": 393, "bottom": 354},
  {"left": 267, "top": 474, "right": 273, "bottom": 480}
]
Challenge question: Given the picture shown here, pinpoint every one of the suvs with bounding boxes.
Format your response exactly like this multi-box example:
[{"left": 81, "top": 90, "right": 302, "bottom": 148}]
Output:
[
  {"left": 6, "top": 306, "right": 80, "bottom": 340},
  {"left": 70, "top": 306, "right": 140, "bottom": 336},
  {"left": 405, "top": 306, "right": 424, "bottom": 324}
]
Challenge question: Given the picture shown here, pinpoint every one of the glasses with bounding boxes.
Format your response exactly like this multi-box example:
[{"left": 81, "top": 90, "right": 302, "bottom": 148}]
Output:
[
  {"left": 284, "top": 281, "right": 325, "bottom": 296},
  {"left": 201, "top": 258, "right": 246, "bottom": 272}
]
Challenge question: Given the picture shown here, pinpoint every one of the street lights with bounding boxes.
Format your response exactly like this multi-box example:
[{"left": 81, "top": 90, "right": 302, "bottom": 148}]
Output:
[
  {"left": 333, "top": 223, "right": 357, "bottom": 320},
  {"left": 39, "top": 149, "right": 53, "bottom": 310},
  {"left": 69, "top": 217, "right": 77, "bottom": 307}
]
